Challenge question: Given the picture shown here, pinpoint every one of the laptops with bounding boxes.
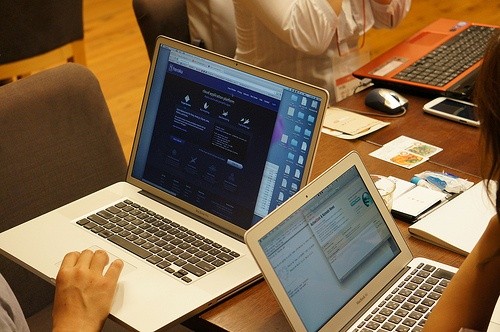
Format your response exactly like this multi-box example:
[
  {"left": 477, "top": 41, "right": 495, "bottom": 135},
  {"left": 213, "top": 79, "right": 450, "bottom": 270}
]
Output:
[
  {"left": 352, "top": 18, "right": 500, "bottom": 99},
  {"left": 244, "top": 149, "right": 458, "bottom": 332},
  {"left": 0, "top": 35, "right": 330, "bottom": 332}
]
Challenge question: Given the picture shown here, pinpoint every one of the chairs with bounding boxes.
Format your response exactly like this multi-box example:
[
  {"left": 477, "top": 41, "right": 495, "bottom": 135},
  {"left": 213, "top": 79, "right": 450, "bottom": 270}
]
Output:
[
  {"left": 132, "top": 0, "right": 237, "bottom": 63},
  {"left": 0, "top": 62, "right": 130, "bottom": 319}
]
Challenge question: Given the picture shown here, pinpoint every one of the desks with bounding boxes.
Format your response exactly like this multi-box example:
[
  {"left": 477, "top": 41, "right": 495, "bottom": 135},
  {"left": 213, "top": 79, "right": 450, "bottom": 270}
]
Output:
[{"left": 184, "top": 75, "right": 500, "bottom": 332}]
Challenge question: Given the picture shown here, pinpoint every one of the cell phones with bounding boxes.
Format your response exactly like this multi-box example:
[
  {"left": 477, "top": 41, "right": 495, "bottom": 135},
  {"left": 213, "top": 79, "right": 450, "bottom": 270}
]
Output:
[{"left": 423, "top": 97, "right": 481, "bottom": 127}]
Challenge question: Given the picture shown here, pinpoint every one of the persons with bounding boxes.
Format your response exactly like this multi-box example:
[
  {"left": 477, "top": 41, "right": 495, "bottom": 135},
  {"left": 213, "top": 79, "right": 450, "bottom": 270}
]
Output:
[
  {"left": 232, "top": 0, "right": 413, "bottom": 107},
  {"left": 419, "top": 28, "right": 500, "bottom": 332},
  {"left": 0, "top": 249, "right": 125, "bottom": 332}
]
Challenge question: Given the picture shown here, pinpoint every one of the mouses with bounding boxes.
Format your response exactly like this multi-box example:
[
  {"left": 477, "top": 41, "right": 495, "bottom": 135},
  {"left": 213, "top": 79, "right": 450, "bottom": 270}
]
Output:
[{"left": 364, "top": 88, "right": 409, "bottom": 115}]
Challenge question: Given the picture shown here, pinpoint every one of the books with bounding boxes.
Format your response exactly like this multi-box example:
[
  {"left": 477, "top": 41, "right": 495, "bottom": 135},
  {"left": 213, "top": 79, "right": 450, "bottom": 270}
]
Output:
[
  {"left": 407, "top": 178, "right": 497, "bottom": 256},
  {"left": 376, "top": 174, "right": 448, "bottom": 227}
]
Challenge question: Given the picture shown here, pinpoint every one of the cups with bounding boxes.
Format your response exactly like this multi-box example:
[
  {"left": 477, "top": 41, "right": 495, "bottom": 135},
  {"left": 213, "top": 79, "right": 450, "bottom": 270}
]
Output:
[{"left": 370, "top": 174, "right": 397, "bottom": 214}]
